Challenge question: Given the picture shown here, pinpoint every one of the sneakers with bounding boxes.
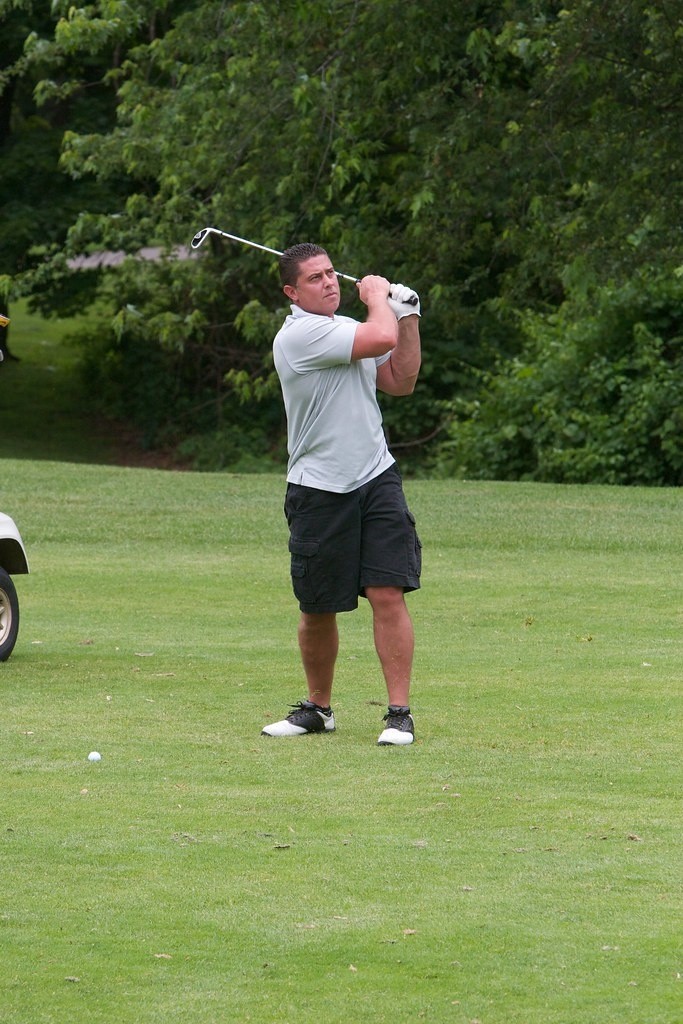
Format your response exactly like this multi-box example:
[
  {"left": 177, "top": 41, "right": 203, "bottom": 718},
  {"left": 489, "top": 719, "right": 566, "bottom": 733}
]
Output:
[
  {"left": 378, "top": 706, "right": 415, "bottom": 745},
  {"left": 261, "top": 701, "right": 335, "bottom": 737}
]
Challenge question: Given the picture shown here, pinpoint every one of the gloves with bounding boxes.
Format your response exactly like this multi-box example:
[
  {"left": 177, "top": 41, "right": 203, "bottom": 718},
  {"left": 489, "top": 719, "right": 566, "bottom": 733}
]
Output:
[{"left": 386, "top": 283, "right": 421, "bottom": 321}]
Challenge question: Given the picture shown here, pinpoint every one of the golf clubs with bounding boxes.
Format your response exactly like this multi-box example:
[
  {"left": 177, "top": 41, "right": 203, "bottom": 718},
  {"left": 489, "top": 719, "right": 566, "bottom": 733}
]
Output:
[{"left": 190, "top": 227, "right": 419, "bottom": 310}]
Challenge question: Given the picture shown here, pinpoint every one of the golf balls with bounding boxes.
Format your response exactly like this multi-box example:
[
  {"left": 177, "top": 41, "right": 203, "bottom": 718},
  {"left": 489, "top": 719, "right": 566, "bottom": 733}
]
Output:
[{"left": 87, "top": 751, "right": 101, "bottom": 762}]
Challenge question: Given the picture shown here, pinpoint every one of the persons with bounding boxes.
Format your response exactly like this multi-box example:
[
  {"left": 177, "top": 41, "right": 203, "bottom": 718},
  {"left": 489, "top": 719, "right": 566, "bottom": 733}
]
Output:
[{"left": 261, "top": 243, "right": 421, "bottom": 745}]
